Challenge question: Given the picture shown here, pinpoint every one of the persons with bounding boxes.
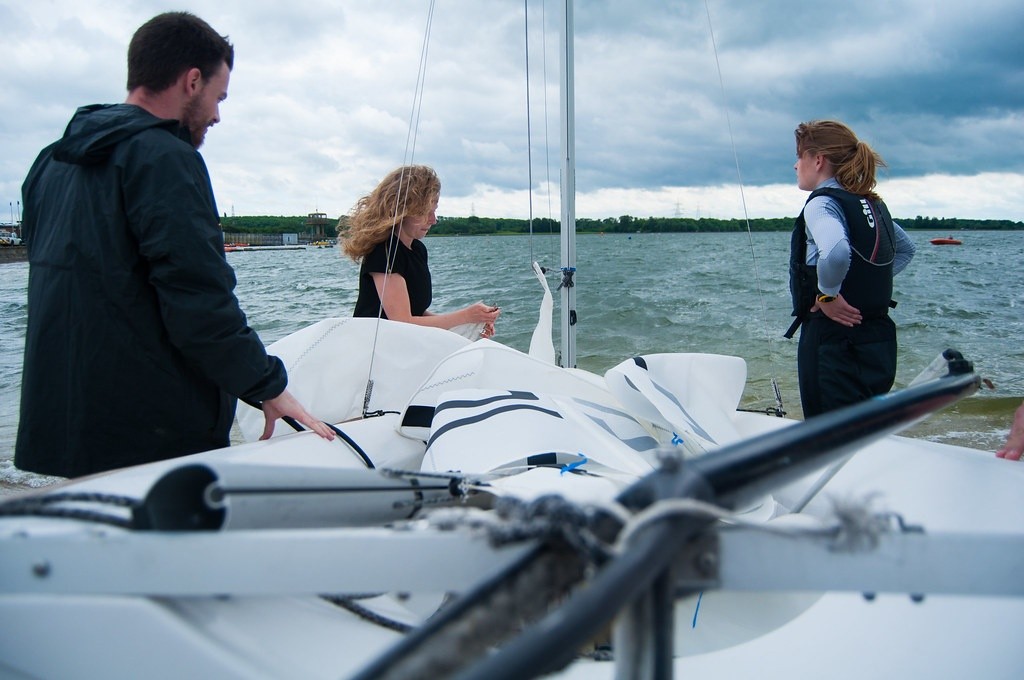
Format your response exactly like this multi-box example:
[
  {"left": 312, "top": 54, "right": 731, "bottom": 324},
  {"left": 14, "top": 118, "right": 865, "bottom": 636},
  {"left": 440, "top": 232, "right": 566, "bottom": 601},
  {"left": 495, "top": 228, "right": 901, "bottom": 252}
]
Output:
[
  {"left": 0, "top": 12, "right": 336, "bottom": 478},
  {"left": 783, "top": 120, "right": 916, "bottom": 420},
  {"left": 335, "top": 164, "right": 501, "bottom": 340},
  {"left": 995, "top": 402, "right": 1024, "bottom": 460}
]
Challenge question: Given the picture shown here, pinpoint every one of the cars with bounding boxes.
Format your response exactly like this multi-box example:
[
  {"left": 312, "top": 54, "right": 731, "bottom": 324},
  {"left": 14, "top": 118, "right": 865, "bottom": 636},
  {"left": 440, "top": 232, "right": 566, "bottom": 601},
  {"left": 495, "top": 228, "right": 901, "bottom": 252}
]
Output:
[{"left": 0, "top": 232, "right": 23, "bottom": 247}]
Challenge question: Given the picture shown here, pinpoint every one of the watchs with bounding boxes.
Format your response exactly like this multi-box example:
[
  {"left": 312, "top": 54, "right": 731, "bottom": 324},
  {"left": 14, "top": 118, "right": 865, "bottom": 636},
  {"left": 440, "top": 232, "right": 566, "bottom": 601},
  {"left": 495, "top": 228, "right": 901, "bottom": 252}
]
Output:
[{"left": 818, "top": 293, "right": 838, "bottom": 304}]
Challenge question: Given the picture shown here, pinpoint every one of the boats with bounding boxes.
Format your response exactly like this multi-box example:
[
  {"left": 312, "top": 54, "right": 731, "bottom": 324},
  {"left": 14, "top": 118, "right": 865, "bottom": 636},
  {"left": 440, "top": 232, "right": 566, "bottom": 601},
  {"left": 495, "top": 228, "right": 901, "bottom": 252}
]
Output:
[
  {"left": 223, "top": 242, "right": 249, "bottom": 251},
  {"left": 929, "top": 235, "right": 961, "bottom": 245}
]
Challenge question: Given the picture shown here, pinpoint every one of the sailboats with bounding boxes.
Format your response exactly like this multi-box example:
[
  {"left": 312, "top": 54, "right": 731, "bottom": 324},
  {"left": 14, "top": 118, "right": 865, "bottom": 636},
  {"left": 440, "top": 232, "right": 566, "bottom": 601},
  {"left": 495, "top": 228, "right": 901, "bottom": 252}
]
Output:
[{"left": 0, "top": 1, "right": 1024, "bottom": 680}]
